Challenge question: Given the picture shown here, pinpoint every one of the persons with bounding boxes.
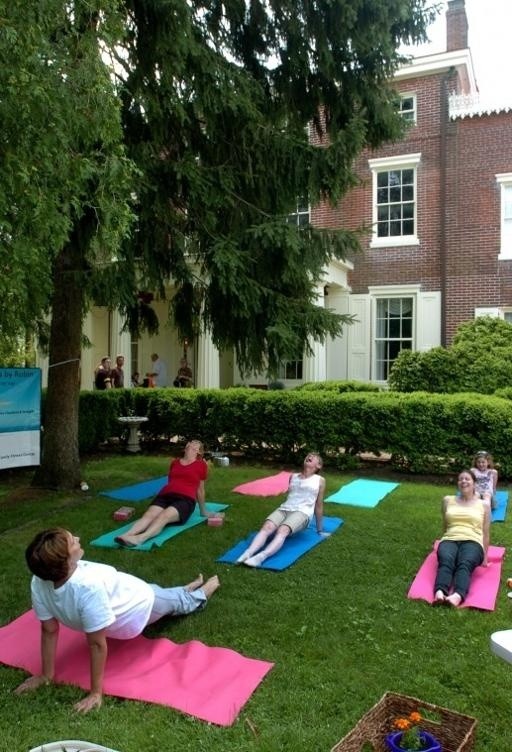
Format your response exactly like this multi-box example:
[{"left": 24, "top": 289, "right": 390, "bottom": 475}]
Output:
[
  {"left": 114, "top": 439, "right": 216, "bottom": 547},
  {"left": 94, "top": 353, "right": 192, "bottom": 388},
  {"left": 431, "top": 469, "right": 490, "bottom": 608},
  {"left": 469, "top": 450, "right": 498, "bottom": 511},
  {"left": 11, "top": 526, "right": 220, "bottom": 716},
  {"left": 233, "top": 452, "right": 331, "bottom": 568}
]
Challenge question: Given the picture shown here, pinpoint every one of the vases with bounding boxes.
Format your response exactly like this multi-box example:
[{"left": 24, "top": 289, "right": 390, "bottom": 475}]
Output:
[{"left": 383, "top": 729, "right": 441, "bottom": 751}]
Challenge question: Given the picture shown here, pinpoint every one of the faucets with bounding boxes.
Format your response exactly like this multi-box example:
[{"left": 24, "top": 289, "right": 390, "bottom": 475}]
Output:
[{"left": 128, "top": 408, "right": 136, "bottom": 417}]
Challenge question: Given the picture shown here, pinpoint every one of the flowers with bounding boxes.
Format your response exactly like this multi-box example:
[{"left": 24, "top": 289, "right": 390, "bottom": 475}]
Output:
[{"left": 392, "top": 711, "right": 423, "bottom": 751}]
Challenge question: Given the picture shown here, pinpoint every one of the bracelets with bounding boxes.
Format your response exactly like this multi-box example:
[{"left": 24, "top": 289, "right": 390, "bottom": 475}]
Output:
[{"left": 317, "top": 530, "right": 322, "bottom": 533}]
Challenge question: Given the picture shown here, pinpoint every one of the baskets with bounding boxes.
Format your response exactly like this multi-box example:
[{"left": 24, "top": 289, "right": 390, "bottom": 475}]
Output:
[{"left": 329, "top": 689, "right": 479, "bottom": 752}]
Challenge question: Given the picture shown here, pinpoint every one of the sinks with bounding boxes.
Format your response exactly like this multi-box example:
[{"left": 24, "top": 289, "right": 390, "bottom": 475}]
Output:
[{"left": 118, "top": 416, "right": 149, "bottom": 426}]
